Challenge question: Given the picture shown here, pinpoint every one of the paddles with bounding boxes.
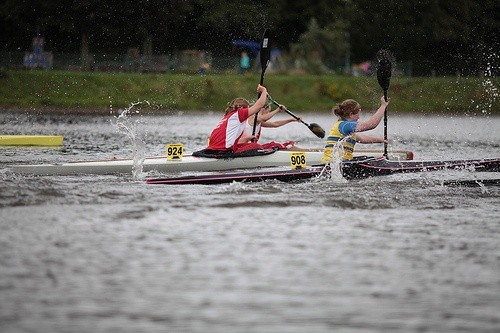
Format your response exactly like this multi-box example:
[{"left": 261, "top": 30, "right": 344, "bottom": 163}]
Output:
[
  {"left": 377, "top": 56, "right": 394, "bottom": 160},
  {"left": 251, "top": 27, "right": 275, "bottom": 144},
  {"left": 264, "top": 92, "right": 326, "bottom": 140}
]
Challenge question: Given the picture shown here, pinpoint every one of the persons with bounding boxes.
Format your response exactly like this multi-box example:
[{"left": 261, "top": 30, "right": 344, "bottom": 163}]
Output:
[
  {"left": 32, "top": 37, "right": 44, "bottom": 68},
  {"left": 321, "top": 95, "right": 391, "bottom": 164},
  {"left": 207, "top": 84, "right": 302, "bottom": 151},
  {"left": 240, "top": 51, "right": 251, "bottom": 74},
  {"left": 199, "top": 62, "right": 209, "bottom": 75}
]
